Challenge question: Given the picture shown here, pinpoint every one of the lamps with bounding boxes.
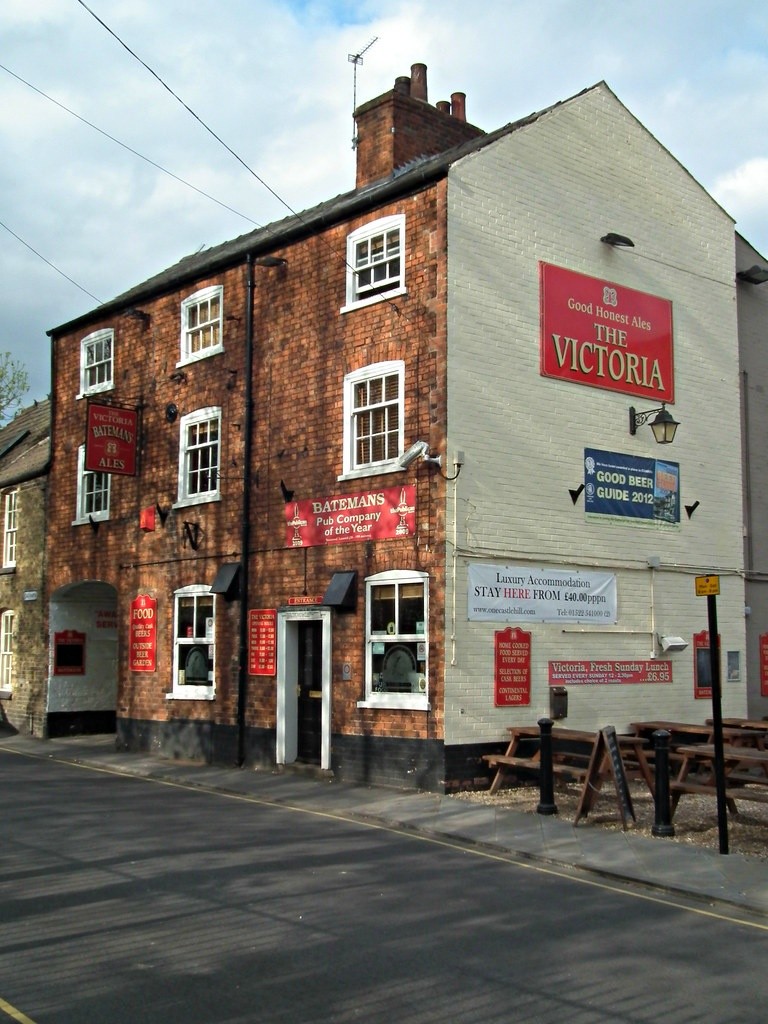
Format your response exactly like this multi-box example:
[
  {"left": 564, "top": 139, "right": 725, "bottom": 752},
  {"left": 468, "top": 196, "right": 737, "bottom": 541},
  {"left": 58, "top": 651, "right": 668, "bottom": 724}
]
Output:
[
  {"left": 737, "top": 262, "right": 768, "bottom": 285},
  {"left": 254, "top": 257, "right": 288, "bottom": 268},
  {"left": 140, "top": 502, "right": 169, "bottom": 533},
  {"left": 629, "top": 401, "right": 681, "bottom": 443},
  {"left": 660, "top": 634, "right": 689, "bottom": 653},
  {"left": 601, "top": 233, "right": 634, "bottom": 248},
  {"left": 131, "top": 309, "right": 150, "bottom": 330}
]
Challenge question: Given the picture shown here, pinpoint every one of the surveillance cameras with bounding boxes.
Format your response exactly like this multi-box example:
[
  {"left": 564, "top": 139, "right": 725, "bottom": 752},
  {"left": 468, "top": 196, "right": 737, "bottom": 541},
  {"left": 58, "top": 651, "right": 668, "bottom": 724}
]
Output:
[{"left": 396, "top": 441, "right": 429, "bottom": 468}]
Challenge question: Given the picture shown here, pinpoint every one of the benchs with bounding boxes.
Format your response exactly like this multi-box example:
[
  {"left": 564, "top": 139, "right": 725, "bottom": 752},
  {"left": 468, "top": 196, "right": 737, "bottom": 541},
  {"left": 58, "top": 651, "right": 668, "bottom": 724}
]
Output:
[
  {"left": 727, "top": 772, "right": 768, "bottom": 784},
  {"left": 670, "top": 782, "right": 768, "bottom": 804},
  {"left": 622, "top": 750, "right": 684, "bottom": 766},
  {"left": 554, "top": 752, "right": 655, "bottom": 769},
  {"left": 482, "top": 755, "right": 588, "bottom": 781}
]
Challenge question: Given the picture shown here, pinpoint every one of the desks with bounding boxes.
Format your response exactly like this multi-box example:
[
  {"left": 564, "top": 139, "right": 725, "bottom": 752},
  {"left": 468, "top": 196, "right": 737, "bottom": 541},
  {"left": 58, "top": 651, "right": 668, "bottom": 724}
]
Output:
[
  {"left": 489, "top": 727, "right": 654, "bottom": 811},
  {"left": 624, "top": 721, "right": 768, "bottom": 773},
  {"left": 705, "top": 718, "right": 768, "bottom": 731},
  {"left": 669, "top": 745, "right": 768, "bottom": 822}
]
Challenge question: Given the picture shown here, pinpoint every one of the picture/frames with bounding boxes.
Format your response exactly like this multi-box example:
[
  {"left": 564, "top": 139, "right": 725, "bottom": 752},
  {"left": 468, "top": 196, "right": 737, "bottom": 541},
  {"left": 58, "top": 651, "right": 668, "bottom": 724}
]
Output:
[{"left": 726, "top": 650, "right": 741, "bottom": 682}]
